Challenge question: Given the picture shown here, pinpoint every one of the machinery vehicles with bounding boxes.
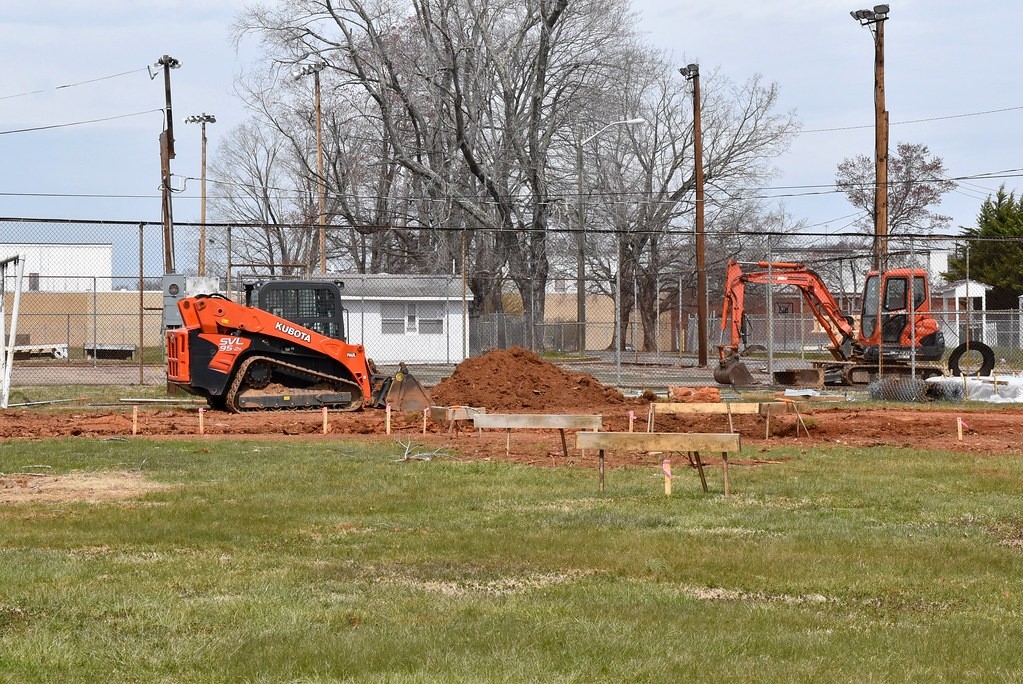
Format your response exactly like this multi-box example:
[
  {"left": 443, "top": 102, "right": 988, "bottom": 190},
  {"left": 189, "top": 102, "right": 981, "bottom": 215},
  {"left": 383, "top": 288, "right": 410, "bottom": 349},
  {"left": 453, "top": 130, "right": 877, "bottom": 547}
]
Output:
[
  {"left": 713, "top": 259, "right": 946, "bottom": 387},
  {"left": 165, "top": 278, "right": 437, "bottom": 415}
]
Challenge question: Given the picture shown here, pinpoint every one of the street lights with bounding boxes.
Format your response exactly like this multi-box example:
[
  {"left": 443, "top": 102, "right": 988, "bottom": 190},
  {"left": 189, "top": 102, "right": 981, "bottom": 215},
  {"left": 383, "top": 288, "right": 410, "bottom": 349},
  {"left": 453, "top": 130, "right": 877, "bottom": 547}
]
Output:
[
  {"left": 184, "top": 113, "right": 216, "bottom": 277},
  {"left": 292, "top": 60, "right": 326, "bottom": 275},
  {"left": 849, "top": 4, "right": 890, "bottom": 271},
  {"left": 157, "top": 54, "right": 184, "bottom": 274},
  {"left": 577, "top": 118, "right": 645, "bottom": 355},
  {"left": 678, "top": 63, "right": 708, "bottom": 369}
]
